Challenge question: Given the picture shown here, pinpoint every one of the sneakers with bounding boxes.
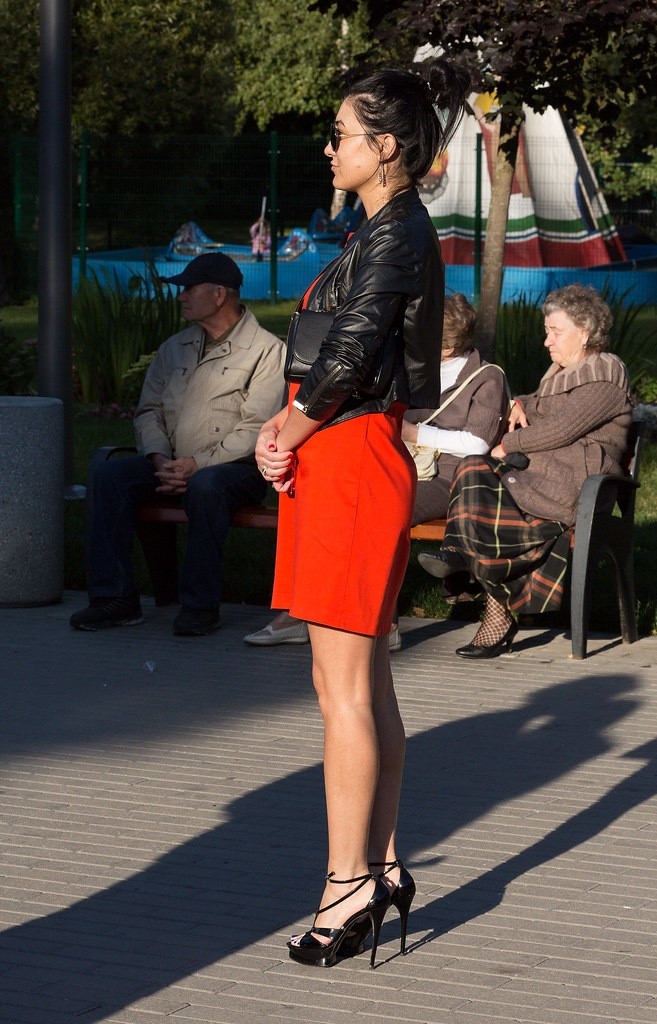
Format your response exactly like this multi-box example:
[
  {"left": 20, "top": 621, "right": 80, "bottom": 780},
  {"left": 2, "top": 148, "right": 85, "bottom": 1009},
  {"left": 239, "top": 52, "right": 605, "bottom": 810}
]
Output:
[
  {"left": 172, "top": 604, "right": 221, "bottom": 636},
  {"left": 70, "top": 594, "right": 144, "bottom": 630}
]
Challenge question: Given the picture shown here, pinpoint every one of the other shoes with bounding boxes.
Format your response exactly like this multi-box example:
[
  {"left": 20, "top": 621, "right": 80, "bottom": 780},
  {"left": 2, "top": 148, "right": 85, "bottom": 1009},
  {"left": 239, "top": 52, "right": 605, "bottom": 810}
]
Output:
[
  {"left": 243, "top": 624, "right": 310, "bottom": 644},
  {"left": 387, "top": 626, "right": 402, "bottom": 650}
]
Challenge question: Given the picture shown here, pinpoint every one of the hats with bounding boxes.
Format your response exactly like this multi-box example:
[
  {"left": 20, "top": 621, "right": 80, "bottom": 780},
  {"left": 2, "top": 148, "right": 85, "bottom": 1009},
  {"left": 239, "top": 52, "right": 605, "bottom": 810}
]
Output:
[{"left": 163, "top": 251, "right": 242, "bottom": 290}]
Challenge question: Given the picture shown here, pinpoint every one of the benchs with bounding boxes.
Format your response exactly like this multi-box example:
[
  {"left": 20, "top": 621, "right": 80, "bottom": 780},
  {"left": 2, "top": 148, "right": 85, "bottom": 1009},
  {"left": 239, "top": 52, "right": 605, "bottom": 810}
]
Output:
[{"left": 89, "top": 413, "right": 649, "bottom": 658}]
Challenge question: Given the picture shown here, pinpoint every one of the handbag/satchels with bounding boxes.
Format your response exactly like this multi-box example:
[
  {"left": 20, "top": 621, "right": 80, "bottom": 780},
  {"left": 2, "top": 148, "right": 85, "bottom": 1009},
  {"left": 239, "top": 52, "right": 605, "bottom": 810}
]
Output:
[{"left": 284, "top": 309, "right": 396, "bottom": 397}]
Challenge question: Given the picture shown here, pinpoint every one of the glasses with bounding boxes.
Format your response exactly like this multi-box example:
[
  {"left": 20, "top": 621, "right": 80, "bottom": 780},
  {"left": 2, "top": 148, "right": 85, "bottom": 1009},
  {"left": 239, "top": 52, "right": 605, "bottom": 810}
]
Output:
[{"left": 330, "top": 124, "right": 406, "bottom": 151}]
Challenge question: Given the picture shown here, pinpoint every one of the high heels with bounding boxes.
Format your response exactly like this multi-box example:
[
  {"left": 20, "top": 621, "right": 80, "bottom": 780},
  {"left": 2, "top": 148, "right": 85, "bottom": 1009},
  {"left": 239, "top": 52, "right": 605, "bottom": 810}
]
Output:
[
  {"left": 363, "top": 860, "right": 417, "bottom": 954},
  {"left": 457, "top": 618, "right": 518, "bottom": 658},
  {"left": 417, "top": 550, "right": 475, "bottom": 583},
  {"left": 286, "top": 871, "right": 391, "bottom": 967}
]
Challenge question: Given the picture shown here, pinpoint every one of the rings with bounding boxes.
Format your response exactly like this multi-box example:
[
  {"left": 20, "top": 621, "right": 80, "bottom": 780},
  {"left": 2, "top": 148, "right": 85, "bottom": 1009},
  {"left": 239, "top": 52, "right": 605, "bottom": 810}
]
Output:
[{"left": 261, "top": 466, "right": 267, "bottom": 475}]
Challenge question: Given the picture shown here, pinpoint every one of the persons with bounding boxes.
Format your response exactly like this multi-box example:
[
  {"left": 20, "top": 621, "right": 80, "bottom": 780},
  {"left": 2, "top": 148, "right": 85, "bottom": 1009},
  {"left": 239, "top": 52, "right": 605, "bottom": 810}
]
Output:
[
  {"left": 383, "top": 292, "right": 512, "bottom": 651},
  {"left": 252, "top": 52, "right": 473, "bottom": 971},
  {"left": 417, "top": 283, "right": 635, "bottom": 659},
  {"left": 67, "top": 251, "right": 288, "bottom": 639}
]
